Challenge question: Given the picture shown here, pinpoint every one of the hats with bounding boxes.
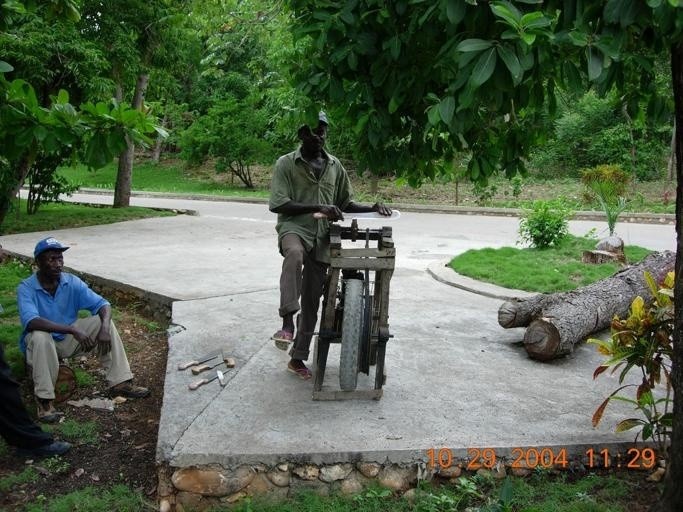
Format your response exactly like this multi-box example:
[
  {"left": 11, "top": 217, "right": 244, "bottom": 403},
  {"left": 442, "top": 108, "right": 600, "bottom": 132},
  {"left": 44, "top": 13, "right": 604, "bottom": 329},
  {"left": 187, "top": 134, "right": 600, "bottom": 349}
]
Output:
[
  {"left": 298, "top": 109, "right": 329, "bottom": 141},
  {"left": 34, "top": 236, "right": 70, "bottom": 258}
]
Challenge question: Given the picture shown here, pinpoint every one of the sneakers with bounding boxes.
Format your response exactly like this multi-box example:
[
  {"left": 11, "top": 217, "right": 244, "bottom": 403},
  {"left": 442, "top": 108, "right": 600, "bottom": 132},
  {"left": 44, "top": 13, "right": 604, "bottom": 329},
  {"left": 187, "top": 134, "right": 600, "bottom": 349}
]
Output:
[
  {"left": 113, "top": 381, "right": 151, "bottom": 399},
  {"left": 34, "top": 395, "right": 58, "bottom": 422}
]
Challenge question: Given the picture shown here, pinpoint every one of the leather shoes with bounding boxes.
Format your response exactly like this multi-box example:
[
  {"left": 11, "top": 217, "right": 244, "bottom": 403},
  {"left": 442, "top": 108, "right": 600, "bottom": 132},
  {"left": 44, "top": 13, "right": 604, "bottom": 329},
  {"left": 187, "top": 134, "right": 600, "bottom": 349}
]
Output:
[{"left": 19, "top": 440, "right": 73, "bottom": 458}]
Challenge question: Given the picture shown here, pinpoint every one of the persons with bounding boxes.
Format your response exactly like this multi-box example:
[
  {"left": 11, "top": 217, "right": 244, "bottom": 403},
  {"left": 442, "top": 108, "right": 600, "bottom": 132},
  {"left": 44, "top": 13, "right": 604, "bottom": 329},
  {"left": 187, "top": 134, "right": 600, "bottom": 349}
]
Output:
[
  {"left": 267, "top": 109, "right": 393, "bottom": 381},
  {"left": 16, "top": 236, "right": 151, "bottom": 424},
  {"left": 0, "top": 302, "right": 74, "bottom": 456}
]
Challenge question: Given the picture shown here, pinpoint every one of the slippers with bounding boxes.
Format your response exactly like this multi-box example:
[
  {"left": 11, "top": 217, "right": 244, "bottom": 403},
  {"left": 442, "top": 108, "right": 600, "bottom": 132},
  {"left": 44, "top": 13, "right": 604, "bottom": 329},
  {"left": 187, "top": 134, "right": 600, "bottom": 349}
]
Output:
[
  {"left": 271, "top": 330, "right": 293, "bottom": 352},
  {"left": 288, "top": 367, "right": 312, "bottom": 380}
]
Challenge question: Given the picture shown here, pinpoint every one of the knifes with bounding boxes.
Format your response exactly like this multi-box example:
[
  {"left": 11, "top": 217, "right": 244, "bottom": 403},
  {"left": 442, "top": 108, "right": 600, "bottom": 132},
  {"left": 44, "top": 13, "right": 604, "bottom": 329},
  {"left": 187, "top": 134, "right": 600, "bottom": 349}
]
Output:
[
  {"left": 216, "top": 368, "right": 225, "bottom": 387},
  {"left": 311, "top": 209, "right": 401, "bottom": 221},
  {"left": 177, "top": 348, "right": 223, "bottom": 370},
  {"left": 189, "top": 358, "right": 224, "bottom": 374},
  {"left": 188, "top": 367, "right": 232, "bottom": 390}
]
